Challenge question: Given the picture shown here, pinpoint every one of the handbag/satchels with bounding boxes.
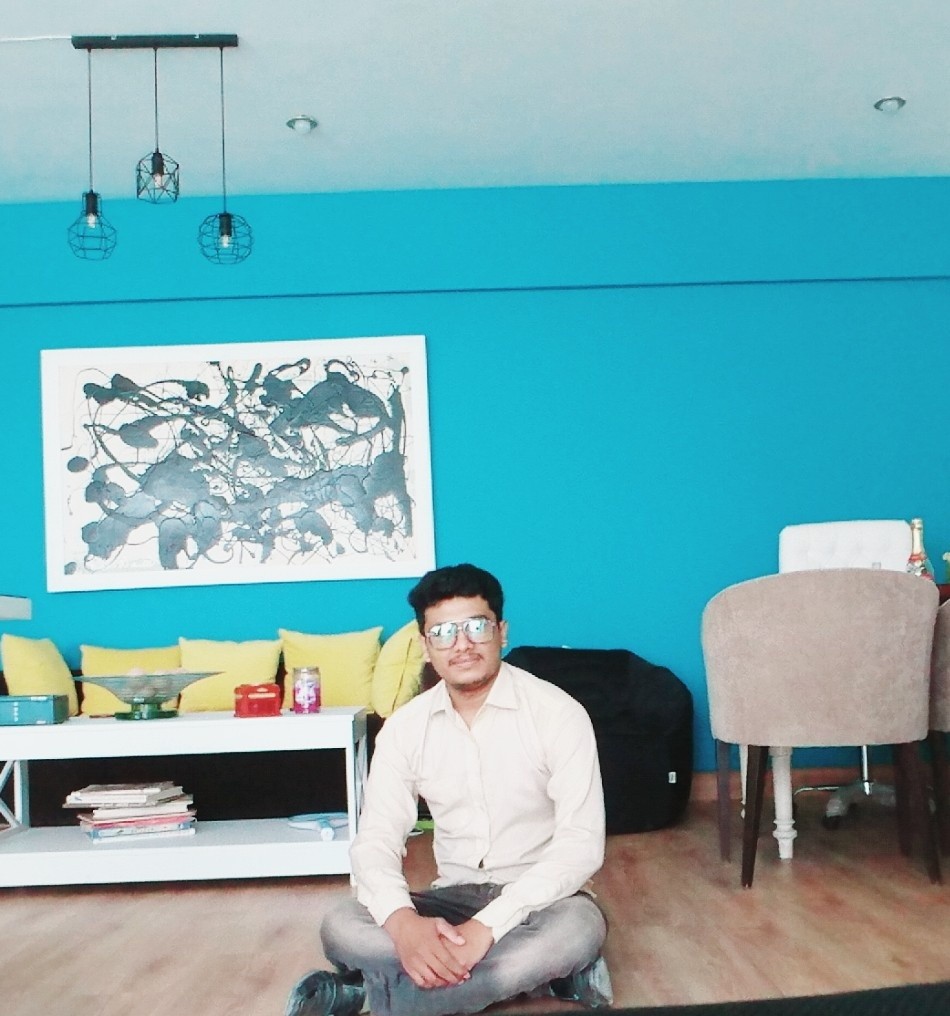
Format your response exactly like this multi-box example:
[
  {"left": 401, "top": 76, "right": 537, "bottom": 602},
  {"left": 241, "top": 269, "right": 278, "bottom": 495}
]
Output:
[{"left": 501, "top": 646, "right": 694, "bottom": 836}]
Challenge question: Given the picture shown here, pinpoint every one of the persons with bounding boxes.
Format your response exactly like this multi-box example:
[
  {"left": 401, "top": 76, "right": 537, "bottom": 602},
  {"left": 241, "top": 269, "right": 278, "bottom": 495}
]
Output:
[{"left": 287, "top": 564, "right": 612, "bottom": 1016}]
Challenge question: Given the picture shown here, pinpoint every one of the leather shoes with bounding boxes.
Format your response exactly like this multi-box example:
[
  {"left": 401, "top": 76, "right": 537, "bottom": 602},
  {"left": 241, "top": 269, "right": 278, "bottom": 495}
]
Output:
[
  {"left": 285, "top": 968, "right": 370, "bottom": 1016},
  {"left": 548, "top": 955, "right": 614, "bottom": 1010}
]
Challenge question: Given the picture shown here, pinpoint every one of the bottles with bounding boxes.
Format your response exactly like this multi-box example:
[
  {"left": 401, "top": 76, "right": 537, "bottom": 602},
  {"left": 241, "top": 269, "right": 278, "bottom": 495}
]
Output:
[
  {"left": 292, "top": 666, "right": 322, "bottom": 715},
  {"left": 907, "top": 519, "right": 936, "bottom": 584}
]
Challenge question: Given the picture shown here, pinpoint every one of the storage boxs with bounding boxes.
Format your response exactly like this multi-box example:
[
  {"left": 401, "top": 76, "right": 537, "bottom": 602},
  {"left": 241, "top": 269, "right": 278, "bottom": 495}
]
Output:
[
  {"left": 0, "top": 694, "right": 69, "bottom": 726},
  {"left": 233, "top": 683, "right": 285, "bottom": 719}
]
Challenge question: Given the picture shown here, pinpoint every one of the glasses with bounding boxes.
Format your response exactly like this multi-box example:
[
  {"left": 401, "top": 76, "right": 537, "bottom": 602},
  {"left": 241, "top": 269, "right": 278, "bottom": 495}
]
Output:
[{"left": 424, "top": 616, "right": 499, "bottom": 650}]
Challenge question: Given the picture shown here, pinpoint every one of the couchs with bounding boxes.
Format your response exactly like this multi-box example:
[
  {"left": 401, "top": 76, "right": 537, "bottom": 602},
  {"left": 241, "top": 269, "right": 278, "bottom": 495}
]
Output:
[
  {"left": 501, "top": 645, "right": 694, "bottom": 838},
  {"left": 0, "top": 670, "right": 434, "bottom": 827}
]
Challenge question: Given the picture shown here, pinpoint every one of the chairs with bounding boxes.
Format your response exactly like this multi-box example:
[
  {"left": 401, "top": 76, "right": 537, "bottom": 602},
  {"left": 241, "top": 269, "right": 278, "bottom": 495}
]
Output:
[
  {"left": 702, "top": 567, "right": 945, "bottom": 893},
  {"left": 779, "top": 518, "right": 936, "bottom": 833},
  {"left": 928, "top": 598, "right": 950, "bottom": 861}
]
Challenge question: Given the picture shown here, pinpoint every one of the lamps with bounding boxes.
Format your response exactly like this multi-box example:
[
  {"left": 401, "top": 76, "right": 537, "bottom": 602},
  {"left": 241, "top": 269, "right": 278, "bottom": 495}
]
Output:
[
  {"left": 67, "top": 32, "right": 256, "bottom": 266},
  {"left": 0, "top": 595, "right": 32, "bottom": 621}
]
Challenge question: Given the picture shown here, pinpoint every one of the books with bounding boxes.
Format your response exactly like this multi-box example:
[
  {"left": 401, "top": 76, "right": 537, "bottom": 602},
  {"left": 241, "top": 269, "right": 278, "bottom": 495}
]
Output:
[{"left": 62, "top": 780, "right": 198, "bottom": 845}]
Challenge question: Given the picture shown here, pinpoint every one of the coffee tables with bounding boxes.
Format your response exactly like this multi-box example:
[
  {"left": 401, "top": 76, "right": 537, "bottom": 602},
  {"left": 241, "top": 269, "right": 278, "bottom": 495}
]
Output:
[{"left": 0, "top": 705, "right": 370, "bottom": 889}]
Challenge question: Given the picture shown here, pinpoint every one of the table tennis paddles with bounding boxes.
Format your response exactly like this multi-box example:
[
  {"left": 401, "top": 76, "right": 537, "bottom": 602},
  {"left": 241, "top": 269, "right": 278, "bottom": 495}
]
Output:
[{"left": 287, "top": 811, "right": 348, "bottom": 842}]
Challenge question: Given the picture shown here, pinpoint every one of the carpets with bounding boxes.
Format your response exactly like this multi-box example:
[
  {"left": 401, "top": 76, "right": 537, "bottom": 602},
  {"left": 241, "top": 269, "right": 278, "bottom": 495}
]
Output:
[{"left": 494, "top": 981, "right": 950, "bottom": 1016}]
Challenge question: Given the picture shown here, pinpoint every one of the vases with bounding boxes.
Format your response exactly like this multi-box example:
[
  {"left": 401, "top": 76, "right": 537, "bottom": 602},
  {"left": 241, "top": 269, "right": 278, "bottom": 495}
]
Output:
[{"left": 906, "top": 517, "right": 935, "bottom": 582}]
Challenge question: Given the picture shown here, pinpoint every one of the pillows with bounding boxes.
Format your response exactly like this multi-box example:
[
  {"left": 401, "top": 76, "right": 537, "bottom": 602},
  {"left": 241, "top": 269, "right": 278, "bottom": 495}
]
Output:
[
  {"left": 177, "top": 635, "right": 282, "bottom": 713},
  {"left": 78, "top": 644, "right": 181, "bottom": 713},
  {"left": 277, "top": 627, "right": 384, "bottom": 714},
  {"left": 2, "top": 633, "right": 81, "bottom": 717},
  {"left": 372, "top": 618, "right": 437, "bottom": 721}
]
